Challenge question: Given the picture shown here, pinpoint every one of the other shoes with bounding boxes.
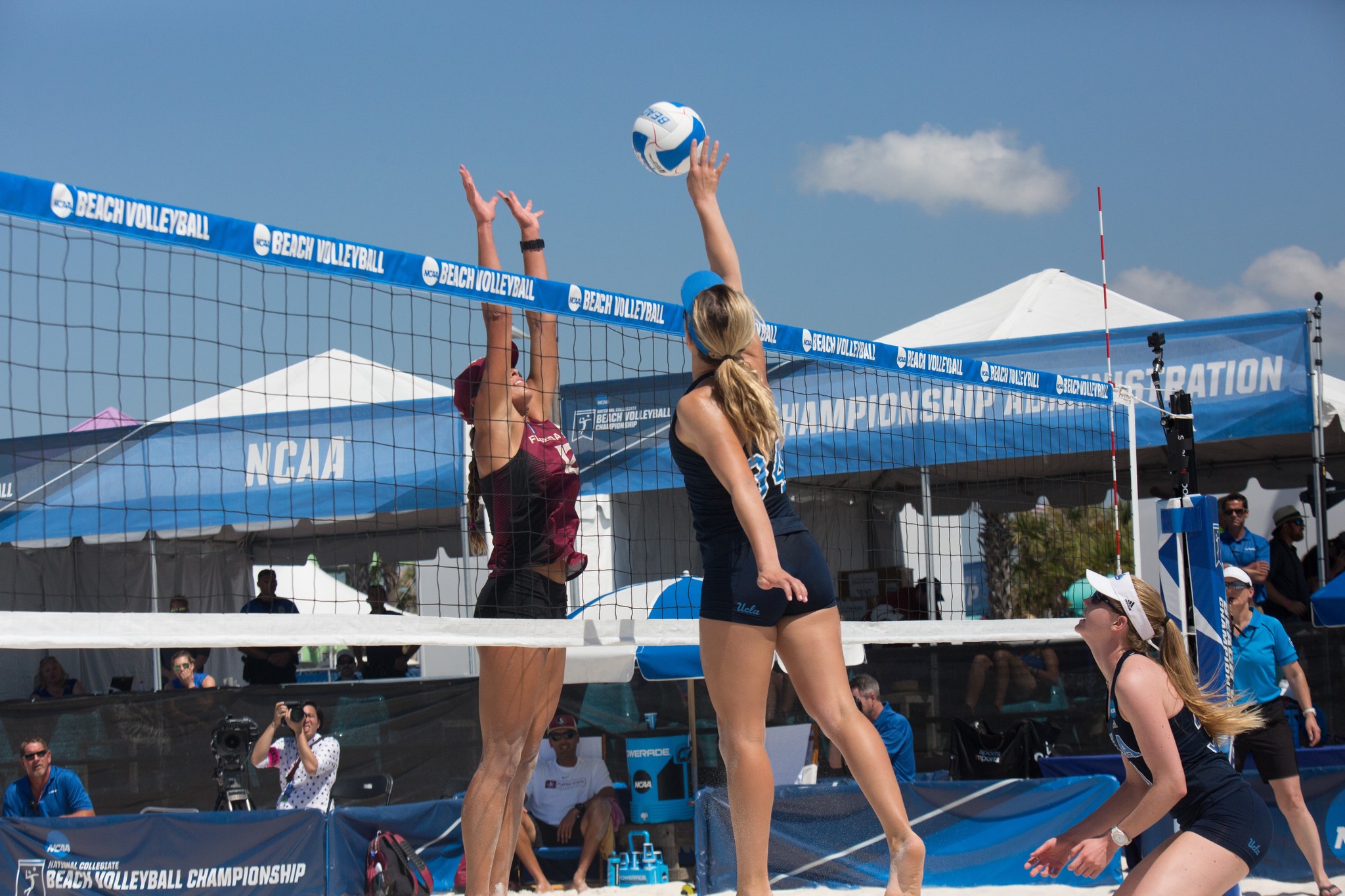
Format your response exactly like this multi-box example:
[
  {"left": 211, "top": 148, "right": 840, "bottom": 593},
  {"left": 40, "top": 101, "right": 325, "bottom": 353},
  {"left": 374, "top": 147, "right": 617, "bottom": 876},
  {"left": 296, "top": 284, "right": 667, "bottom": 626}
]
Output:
[{"left": 956, "top": 703, "right": 1002, "bottom": 719}]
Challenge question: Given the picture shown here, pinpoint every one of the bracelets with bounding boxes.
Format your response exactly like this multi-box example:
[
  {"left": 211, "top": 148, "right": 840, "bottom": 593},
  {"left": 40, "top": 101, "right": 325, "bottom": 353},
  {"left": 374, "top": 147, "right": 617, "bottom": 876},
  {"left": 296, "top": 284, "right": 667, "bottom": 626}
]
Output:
[
  {"left": 519, "top": 239, "right": 545, "bottom": 253},
  {"left": 575, "top": 803, "right": 588, "bottom": 816}
]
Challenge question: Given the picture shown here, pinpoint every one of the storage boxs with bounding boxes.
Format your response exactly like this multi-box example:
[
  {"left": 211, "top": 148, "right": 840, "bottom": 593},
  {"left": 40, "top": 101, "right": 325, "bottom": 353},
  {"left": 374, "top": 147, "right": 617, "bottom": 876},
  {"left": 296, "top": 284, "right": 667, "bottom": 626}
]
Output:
[{"left": 836, "top": 568, "right": 913, "bottom": 622}]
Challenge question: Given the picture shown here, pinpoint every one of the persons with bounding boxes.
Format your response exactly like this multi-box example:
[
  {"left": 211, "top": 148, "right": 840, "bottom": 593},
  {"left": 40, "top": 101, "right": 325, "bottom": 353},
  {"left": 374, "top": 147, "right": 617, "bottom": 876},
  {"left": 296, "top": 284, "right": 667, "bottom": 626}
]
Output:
[
  {"left": 959, "top": 612, "right": 1061, "bottom": 726},
  {"left": 1218, "top": 494, "right": 1345, "bottom": 896},
  {"left": 2, "top": 735, "right": 96, "bottom": 818},
  {"left": 849, "top": 674, "right": 917, "bottom": 782},
  {"left": 1023, "top": 571, "right": 1272, "bottom": 896},
  {"left": 449, "top": 165, "right": 587, "bottom": 896},
  {"left": 667, "top": 136, "right": 925, "bottom": 896},
  {"left": 159, "top": 595, "right": 211, "bottom": 685},
  {"left": 332, "top": 649, "right": 360, "bottom": 681},
  {"left": 514, "top": 713, "right": 614, "bottom": 893},
  {"left": 238, "top": 568, "right": 303, "bottom": 686},
  {"left": 163, "top": 650, "right": 215, "bottom": 691},
  {"left": 352, "top": 583, "right": 421, "bottom": 679},
  {"left": 28, "top": 658, "right": 86, "bottom": 701},
  {"left": 251, "top": 700, "right": 340, "bottom": 814},
  {"left": 867, "top": 576, "right": 944, "bottom": 657}
]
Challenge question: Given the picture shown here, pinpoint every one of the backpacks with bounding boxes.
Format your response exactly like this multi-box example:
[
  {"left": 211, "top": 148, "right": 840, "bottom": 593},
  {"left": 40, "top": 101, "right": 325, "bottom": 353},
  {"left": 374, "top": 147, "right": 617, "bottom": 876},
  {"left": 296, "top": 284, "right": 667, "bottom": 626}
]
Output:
[{"left": 364, "top": 830, "right": 434, "bottom": 896}]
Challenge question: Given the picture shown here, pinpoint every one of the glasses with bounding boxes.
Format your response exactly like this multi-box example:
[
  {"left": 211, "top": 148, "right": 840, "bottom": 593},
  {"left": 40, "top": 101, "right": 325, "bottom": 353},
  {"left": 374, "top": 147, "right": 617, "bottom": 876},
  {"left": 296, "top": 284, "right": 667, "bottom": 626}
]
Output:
[
  {"left": 173, "top": 663, "right": 190, "bottom": 671},
  {"left": 1091, "top": 590, "right": 1135, "bottom": 633},
  {"left": 1223, "top": 508, "right": 1248, "bottom": 515},
  {"left": 1224, "top": 581, "right": 1248, "bottom": 591},
  {"left": 683, "top": 309, "right": 692, "bottom": 338},
  {"left": 1286, "top": 519, "right": 1304, "bottom": 526},
  {"left": 339, "top": 660, "right": 354, "bottom": 665},
  {"left": 170, "top": 607, "right": 187, "bottom": 614},
  {"left": 550, "top": 730, "right": 576, "bottom": 741},
  {"left": 21, "top": 750, "right": 47, "bottom": 760}
]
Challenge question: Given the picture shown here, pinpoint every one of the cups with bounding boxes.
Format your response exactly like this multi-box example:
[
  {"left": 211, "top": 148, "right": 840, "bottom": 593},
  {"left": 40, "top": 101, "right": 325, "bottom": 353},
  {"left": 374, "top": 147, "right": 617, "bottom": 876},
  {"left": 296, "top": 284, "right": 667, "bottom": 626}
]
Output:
[{"left": 644, "top": 712, "right": 658, "bottom": 729}]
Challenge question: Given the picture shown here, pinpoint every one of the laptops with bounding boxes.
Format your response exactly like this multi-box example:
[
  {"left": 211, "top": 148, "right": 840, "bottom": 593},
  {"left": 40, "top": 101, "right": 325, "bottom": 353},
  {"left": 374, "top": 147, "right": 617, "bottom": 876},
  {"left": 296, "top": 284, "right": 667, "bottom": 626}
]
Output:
[{"left": 107, "top": 676, "right": 133, "bottom": 695}]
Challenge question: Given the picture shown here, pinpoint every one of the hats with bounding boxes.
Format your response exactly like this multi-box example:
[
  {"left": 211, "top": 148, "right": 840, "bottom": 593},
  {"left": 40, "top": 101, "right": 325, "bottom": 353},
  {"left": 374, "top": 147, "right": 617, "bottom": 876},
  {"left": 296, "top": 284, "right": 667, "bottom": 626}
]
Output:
[
  {"left": 454, "top": 339, "right": 519, "bottom": 425},
  {"left": 870, "top": 604, "right": 902, "bottom": 621},
  {"left": 545, "top": 715, "right": 578, "bottom": 735},
  {"left": 681, "top": 270, "right": 726, "bottom": 356},
  {"left": 1086, "top": 569, "right": 1161, "bottom": 651},
  {"left": 1271, "top": 506, "right": 1309, "bottom": 535},
  {"left": 918, "top": 577, "right": 944, "bottom": 602},
  {"left": 336, "top": 649, "right": 355, "bottom": 663},
  {"left": 1222, "top": 566, "right": 1252, "bottom": 588}
]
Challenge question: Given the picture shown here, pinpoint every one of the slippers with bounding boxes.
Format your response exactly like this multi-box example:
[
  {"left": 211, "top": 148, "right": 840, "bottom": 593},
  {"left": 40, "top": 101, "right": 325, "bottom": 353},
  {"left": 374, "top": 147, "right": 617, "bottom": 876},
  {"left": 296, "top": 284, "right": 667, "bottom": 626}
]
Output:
[{"left": 1317, "top": 884, "right": 1343, "bottom": 896}]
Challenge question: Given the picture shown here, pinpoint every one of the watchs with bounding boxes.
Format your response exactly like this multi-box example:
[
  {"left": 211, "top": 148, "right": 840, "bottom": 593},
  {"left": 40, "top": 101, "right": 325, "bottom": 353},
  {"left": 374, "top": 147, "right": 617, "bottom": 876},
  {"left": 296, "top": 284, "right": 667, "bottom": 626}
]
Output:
[
  {"left": 1301, "top": 708, "right": 1316, "bottom": 717},
  {"left": 1110, "top": 824, "right": 1131, "bottom": 847}
]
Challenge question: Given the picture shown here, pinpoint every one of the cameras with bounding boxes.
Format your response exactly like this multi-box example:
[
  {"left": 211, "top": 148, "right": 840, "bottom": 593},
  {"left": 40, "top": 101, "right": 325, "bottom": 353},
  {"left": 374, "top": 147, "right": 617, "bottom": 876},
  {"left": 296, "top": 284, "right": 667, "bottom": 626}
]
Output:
[
  {"left": 1147, "top": 332, "right": 1165, "bottom": 348},
  {"left": 281, "top": 701, "right": 304, "bottom": 727}
]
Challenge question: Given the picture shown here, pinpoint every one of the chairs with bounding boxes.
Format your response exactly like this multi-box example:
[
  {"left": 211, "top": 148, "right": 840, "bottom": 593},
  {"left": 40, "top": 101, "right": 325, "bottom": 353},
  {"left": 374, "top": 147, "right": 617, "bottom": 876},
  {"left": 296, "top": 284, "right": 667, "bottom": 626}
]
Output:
[
  {"left": 326, "top": 773, "right": 393, "bottom": 814},
  {"left": 516, "top": 735, "right": 626, "bottom": 892}
]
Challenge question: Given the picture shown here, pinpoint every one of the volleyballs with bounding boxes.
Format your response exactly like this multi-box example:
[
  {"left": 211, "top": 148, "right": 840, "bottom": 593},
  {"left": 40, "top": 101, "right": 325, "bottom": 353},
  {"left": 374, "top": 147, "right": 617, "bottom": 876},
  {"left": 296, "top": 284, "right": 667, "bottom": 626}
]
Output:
[{"left": 632, "top": 101, "right": 707, "bottom": 177}]
